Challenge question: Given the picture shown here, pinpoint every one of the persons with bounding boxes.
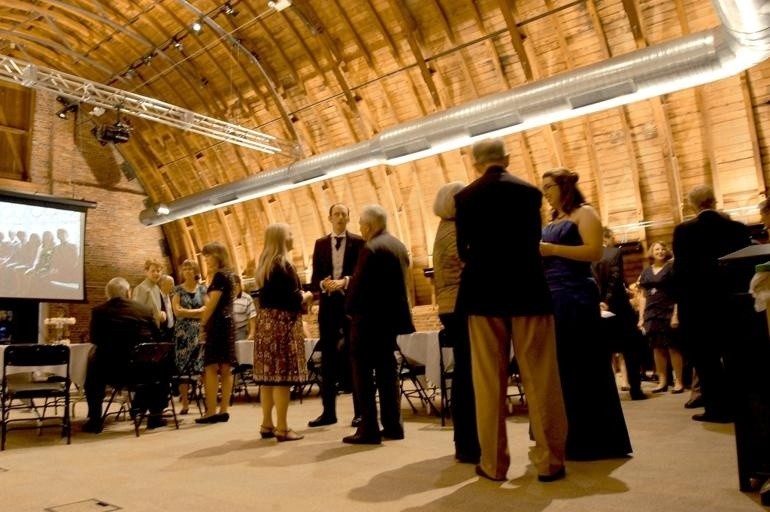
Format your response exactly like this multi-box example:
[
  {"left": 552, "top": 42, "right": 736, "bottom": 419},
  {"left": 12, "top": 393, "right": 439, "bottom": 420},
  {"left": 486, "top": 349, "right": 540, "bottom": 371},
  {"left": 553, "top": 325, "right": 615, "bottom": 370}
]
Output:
[{"left": 0, "top": 229, "right": 78, "bottom": 295}]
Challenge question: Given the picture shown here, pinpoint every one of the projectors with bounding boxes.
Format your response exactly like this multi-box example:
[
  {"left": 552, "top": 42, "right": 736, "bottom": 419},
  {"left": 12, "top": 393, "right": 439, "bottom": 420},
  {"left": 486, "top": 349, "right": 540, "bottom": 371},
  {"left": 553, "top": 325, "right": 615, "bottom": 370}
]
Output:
[{"left": 102, "top": 129, "right": 130, "bottom": 143}]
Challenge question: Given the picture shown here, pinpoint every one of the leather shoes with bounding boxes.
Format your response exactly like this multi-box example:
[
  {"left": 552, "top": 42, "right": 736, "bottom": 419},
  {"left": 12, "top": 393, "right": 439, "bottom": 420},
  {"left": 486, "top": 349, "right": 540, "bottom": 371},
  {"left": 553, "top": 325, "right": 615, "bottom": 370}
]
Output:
[
  {"left": 693, "top": 413, "right": 709, "bottom": 421},
  {"left": 343, "top": 417, "right": 404, "bottom": 444},
  {"left": 309, "top": 416, "right": 337, "bottom": 426},
  {"left": 652, "top": 385, "right": 684, "bottom": 393},
  {"left": 82, "top": 422, "right": 103, "bottom": 433},
  {"left": 148, "top": 419, "right": 167, "bottom": 428},
  {"left": 195, "top": 413, "right": 228, "bottom": 423}
]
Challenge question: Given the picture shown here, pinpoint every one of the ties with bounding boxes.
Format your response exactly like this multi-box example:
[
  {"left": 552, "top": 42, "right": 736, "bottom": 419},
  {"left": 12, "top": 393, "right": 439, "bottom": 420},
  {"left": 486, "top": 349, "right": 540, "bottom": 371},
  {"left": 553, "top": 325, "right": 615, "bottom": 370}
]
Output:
[{"left": 334, "top": 237, "right": 343, "bottom": 250}]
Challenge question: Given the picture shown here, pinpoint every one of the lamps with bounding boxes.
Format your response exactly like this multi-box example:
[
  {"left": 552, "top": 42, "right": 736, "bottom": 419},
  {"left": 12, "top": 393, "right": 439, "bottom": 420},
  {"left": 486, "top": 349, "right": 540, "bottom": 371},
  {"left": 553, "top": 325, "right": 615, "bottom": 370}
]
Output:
[
  {"left": 88, "top": 96, "right": 130, "bottom": 147},
  {"left": 50, "top": 90, "right": 78, "bottom": 123},
  {"left": 105, "top": 0, "right": 248, "bottom": 86}
]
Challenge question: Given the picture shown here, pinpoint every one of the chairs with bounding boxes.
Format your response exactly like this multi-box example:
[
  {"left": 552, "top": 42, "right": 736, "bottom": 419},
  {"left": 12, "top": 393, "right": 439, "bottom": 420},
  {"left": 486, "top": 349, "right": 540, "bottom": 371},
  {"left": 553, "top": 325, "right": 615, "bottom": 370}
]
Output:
[
  {"left": 231, "top": 329, "right": 528, "bottom": 427},
  {"left": 0, "top": 309, "right": 205, "bottom": 450}
]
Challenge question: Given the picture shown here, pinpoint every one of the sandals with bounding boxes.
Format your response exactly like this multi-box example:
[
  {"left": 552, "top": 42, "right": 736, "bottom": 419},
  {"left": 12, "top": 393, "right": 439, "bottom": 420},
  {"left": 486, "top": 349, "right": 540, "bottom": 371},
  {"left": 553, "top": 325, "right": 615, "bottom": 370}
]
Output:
[{"left": 260, "top": 425, "right": 303, "bottom": 441}]
